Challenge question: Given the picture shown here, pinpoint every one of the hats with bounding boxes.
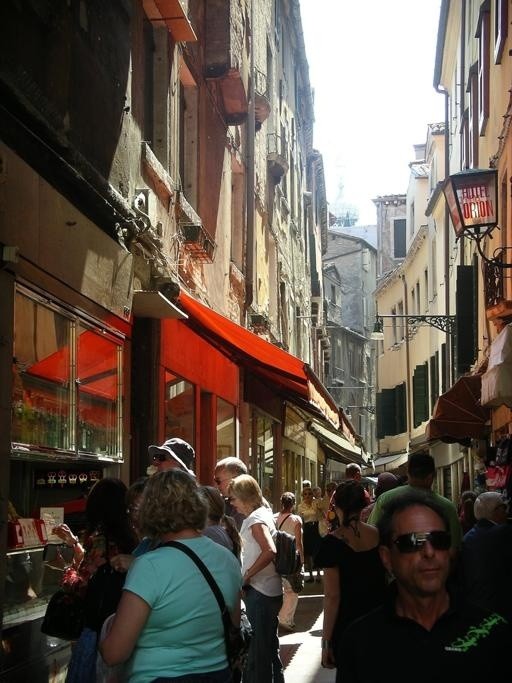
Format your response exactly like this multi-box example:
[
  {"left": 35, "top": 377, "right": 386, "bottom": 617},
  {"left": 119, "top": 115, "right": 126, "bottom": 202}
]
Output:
[{"left": 148, "top": 438, "right": 196, "bottom": 477}]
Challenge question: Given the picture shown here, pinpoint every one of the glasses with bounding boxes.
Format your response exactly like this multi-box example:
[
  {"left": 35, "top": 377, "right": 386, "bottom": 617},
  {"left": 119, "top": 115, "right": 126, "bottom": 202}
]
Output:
[
  {"left": 395, "top": 530, "right": 452, "bottom": 553},
  {"left": 152, "top": 453, "right": 166, "bottom": 461},
  {"left": 213, "top": 476, "right": 233, "bottom": 484}
]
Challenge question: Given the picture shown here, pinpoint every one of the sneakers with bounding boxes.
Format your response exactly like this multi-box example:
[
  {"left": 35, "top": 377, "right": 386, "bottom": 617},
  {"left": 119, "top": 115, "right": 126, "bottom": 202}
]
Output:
[
  {"left": 279, "top": 623, "right": 295, "bottom": 632},
  {"left": 305, "top": 579, "right": 321, "bottom": 583}
]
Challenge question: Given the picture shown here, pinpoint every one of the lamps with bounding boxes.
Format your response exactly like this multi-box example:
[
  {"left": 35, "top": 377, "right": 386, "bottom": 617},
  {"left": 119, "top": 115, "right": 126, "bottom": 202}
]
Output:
[{"left": 441, "top": 165, "right": 512, "bottom": 280}]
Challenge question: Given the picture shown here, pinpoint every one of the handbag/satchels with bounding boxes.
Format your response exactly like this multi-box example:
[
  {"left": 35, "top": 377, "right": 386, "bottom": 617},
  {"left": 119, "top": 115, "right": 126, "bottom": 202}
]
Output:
[
  {"left": 40, "top": 587, "right": 83, "bottom": 640},
  {"left": 83, "top": 563, "right": 121, "bottom": 631},
  {"left": 274, "top": 531, "right": 304, "bottom": 593},
  {"left": 225, "top": 609, "right": 254, "bottom": 683},
  {"left": 485, "top": 434, "right": 510, "bottom": 488}
]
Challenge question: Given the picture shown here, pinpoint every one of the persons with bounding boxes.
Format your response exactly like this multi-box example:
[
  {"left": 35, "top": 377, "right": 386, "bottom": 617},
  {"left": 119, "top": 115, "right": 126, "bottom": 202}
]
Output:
[
  {"left": 50, "top": 437, "right": 335, "bottom": 683},
  {"left": 312, "top": 453, "right": 511, "bottom": 680}
]
